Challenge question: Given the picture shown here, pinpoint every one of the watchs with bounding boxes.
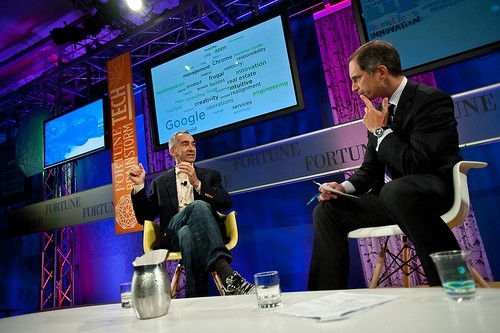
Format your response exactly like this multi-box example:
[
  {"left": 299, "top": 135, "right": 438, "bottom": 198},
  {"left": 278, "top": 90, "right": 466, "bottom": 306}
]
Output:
[{"left": 373, "top": 126, "right": 391, "bottom": 137}]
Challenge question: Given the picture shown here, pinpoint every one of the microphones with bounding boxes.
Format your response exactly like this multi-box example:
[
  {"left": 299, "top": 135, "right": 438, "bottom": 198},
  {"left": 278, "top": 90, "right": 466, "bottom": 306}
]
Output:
[{"left": 181, "top": 181, "right": 187, "bottom": 186}]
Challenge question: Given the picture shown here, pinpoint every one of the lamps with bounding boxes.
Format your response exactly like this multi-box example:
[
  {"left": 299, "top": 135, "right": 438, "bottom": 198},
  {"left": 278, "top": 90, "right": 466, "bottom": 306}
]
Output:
[{"left": 48, "top": 1, "right": 147, "bottom": 46}]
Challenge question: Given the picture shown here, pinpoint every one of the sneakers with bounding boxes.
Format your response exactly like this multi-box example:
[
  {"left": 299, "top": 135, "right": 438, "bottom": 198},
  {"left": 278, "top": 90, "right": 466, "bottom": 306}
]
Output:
[{"left": 222, "top": 271, "right": 255, "bottom": 296}]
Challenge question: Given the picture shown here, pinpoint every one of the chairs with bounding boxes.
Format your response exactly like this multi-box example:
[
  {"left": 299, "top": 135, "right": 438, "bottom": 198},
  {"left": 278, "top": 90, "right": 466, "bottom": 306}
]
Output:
[
  {"left": 348, "top": 161, "right": 490, "bottom": 290},
  {"left": 143, "top": 211, "right": 239, "bottom": 300}
]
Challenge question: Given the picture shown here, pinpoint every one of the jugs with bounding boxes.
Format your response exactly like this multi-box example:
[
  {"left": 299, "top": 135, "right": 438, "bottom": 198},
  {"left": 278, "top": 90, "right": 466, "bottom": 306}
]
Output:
[{"left": 131, "top": 249, "right": 171, "bottom": 320}]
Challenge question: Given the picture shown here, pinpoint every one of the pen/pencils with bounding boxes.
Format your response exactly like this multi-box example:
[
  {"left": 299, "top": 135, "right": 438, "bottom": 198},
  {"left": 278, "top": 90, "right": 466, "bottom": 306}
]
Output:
[{"left": 306, "top": 192, "right": 321, "bottom": 205}]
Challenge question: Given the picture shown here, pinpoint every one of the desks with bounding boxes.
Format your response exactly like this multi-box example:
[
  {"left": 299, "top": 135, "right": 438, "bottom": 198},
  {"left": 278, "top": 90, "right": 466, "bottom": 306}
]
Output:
[{"left": 0, "top": 286, "right": 500, "bottom": 333}]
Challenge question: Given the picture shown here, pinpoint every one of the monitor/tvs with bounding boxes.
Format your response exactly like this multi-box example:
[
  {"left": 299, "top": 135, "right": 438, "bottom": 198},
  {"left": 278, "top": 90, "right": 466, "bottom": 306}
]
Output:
[
  {"left": 43, "top": 96, "right": 106, "bottom": 169},
  {"left": 146, "top": 7, "right": 305, "bottom": 152}
]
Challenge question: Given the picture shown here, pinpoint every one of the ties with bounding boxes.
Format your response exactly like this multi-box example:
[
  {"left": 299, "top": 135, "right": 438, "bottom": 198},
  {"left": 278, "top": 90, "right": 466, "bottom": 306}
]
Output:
[{"left": 386, "top": 104, "right": 396, "bottom": 127}]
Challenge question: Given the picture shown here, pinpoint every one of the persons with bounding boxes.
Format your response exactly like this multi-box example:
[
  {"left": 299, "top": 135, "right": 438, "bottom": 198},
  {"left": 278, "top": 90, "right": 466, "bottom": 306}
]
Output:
[
  {"left": 128, "top": 131, "right": 257, "bottom": 298},
  {"left": 307, "top": 41, "right": 471, "bottom": 288}
]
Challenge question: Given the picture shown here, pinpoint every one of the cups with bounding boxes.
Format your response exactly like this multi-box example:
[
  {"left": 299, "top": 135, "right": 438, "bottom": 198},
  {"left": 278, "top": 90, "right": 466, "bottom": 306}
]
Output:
[
  {"left": 430, "top": 250, "right": 476, "bottom": 301},
  {"left": 120, "top": 282, "right": 132, "bottom": 309},
  {"left": 254, "top": 271, "right": 283, "bottom": 309}
]
